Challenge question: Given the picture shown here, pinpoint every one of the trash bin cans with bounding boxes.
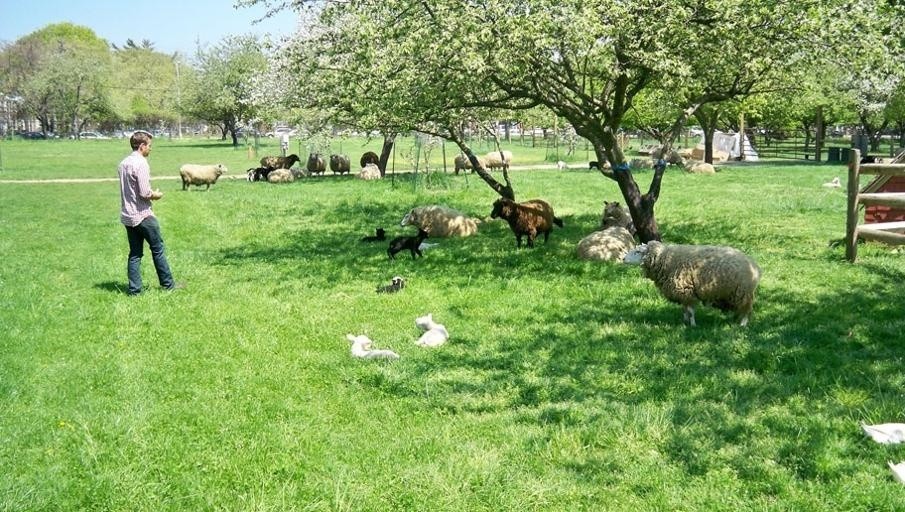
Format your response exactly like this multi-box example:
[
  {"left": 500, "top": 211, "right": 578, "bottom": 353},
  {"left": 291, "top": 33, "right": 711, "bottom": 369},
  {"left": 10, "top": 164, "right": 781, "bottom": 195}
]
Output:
[{"left": 829, "top": 147, "right": 849, "bottom": 163}]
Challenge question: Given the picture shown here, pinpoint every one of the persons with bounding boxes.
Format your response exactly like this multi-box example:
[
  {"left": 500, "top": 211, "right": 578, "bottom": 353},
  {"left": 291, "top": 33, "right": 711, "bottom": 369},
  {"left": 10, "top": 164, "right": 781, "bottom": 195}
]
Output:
[
  {"left": 118, "top": 131, "right": 175, "bottom": 296},
  {"left": 279, "top": 132, "right": 289, "bottom": 157},
  {"left": 567, "top": 137, "right": 577, "bottom": 156}
]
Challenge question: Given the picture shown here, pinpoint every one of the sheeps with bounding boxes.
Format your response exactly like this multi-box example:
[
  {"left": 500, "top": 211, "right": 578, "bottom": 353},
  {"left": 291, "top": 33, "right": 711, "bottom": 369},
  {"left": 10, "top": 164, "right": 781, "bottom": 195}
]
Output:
[
  {"left": 822, "top": 177, "right": 841, "bottom": 188},
  {"left": 589, "top": 161, "right": 598, "bottom": 170},
  {"left": 387, "top": 228, "right": 429, "bottom": 260},
  {"left": 414, "top": 313, "right": 449, "bottom": 347},
  {"left": 491, "top": 198, "right": 564, "bottom": 249},
  {"left": 623, "top": 240, "right": 761, "bottom": 329},
  {"left": 629, "top": 158, "right": 654, "bottom": 170},
  {"left": 376, "top": 276, "right": 405, "bottom": 294},
  {"left": 558, "top": 161, "right": 568, "bottom": 169},
  {"left": 681, "top": 157, "right": 716, "bottom": 174},
  {"left": 453, "top": 151, "right": 512, "bottom": 175},
  {"left": 180, "top": 164, "right": 228, "bottom": 192},
  {"left": 601, "top": 201, "right": 638, "bottom": 237},
  {"left": 246, "top": 153, "right": 349, "bottom": 183},
  {"left": 401, "top": 205, "right": 483, "bottom": 238},
  {"left": 576, "top": 226, "right": 635, "bottom": 263},
  {"left": 346, "top": 333, "right": 399, "bottom": 359},
  {"left": 362, "top": 228, "right": 386, "bottom": 243},
  {"left": 360, "top": 152, "right": 384, "bottom": 180}
]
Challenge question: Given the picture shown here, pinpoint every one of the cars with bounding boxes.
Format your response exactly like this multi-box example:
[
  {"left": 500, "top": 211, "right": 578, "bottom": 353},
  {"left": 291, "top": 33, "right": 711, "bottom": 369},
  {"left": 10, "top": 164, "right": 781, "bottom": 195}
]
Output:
[
  {"left": 24, "top": 131, "right": 112, "bottom": 139},
  {"left": 266, "top": 128, "right": 292, "bottom": 139}
]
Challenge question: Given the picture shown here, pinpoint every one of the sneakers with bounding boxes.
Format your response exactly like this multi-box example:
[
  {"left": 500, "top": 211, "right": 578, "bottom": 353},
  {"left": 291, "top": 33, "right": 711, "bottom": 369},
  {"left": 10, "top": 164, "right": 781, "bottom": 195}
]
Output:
[{"left": 176, "top": 284, "right": 186, "bottom": 288}]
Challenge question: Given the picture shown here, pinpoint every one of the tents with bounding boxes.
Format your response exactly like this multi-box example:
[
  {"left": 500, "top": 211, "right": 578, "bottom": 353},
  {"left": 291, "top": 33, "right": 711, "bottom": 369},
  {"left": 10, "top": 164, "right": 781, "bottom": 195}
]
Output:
[{"left": 699, "top": 131, "right": 758, "bottom": 161}]
